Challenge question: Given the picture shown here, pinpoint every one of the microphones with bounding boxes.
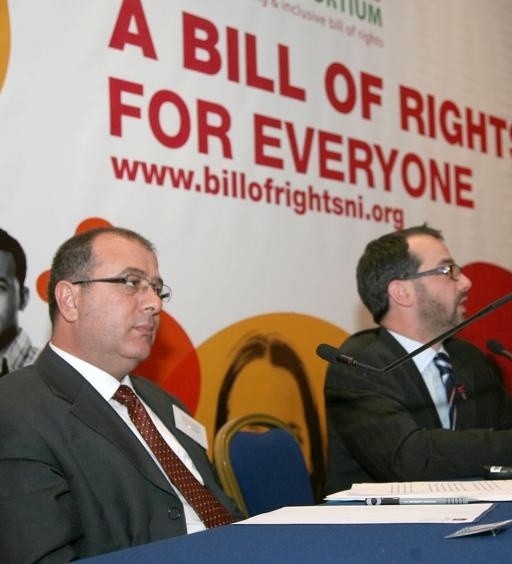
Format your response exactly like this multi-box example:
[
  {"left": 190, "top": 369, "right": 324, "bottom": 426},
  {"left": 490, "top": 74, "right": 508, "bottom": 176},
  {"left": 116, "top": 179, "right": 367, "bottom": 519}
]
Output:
[
  {"left": 485, "top": 337, "right": 512, "bottom": 362},
  {"left": 315, "top": 292, "right": 512, "bottom": 374}
]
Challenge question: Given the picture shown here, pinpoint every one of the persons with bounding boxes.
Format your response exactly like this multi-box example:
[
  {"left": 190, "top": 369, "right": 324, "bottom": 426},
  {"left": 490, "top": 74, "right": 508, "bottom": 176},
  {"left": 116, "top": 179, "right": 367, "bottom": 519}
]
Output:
[
  {"left": 0, "top": 228, "right": 44, "bottom": 377},
  {"left": 324, "top": 225, "right": 511, "bottom": 494},
  {"left": 0, "top": 228, "right": 247, "bottom": 564},
  {"left": 215, "top": 332, "right": 326, "bottom": 504}
]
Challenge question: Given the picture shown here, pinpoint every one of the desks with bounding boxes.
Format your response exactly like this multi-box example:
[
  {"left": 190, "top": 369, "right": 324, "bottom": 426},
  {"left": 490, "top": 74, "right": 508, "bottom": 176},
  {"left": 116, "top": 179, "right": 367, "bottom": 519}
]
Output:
[{"left": 69, "top": 480, "right": 512, "bottom": 563}]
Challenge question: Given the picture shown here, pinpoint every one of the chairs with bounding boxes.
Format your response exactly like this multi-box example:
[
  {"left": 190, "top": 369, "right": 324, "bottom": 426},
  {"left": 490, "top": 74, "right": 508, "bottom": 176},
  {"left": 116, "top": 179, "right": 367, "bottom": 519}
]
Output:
[{"left": 212, "top": 411, "right": 318, "bottom": 516}]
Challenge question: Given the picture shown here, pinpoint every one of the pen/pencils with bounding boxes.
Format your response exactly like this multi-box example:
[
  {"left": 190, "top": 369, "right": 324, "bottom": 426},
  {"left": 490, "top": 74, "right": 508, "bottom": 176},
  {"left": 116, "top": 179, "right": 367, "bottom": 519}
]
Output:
[{"left": 364, "top": 497, "right": 480, "bottom": 505}]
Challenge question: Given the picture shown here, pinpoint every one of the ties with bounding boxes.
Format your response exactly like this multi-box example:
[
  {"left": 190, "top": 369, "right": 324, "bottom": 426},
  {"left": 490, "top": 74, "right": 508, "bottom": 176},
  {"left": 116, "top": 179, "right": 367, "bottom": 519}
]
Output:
[
  {"left": 433, "top": 351, "right": 462, "bottom": 433},
  {"left": 112, "top": 383, "right": 238, "bottom": 531}
]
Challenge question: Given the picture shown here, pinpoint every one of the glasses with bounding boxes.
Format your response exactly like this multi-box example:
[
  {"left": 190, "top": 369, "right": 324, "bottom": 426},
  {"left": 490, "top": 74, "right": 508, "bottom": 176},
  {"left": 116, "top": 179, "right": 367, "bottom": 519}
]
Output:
[
  {"left": 72, "top": 273, "right": 174, "bottom": 305},
  {"left": 385, "top": 264, "right": 463, "bottom": 287}
]
